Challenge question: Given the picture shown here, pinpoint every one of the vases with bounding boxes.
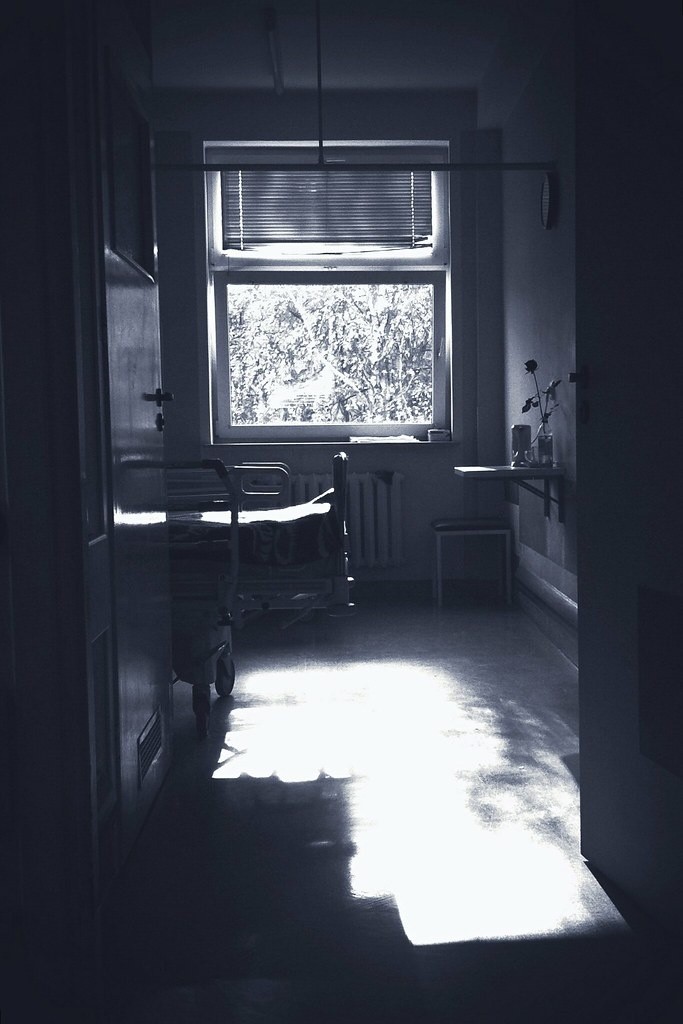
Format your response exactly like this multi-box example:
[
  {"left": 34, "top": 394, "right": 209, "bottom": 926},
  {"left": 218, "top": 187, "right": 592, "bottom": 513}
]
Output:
[{"left": 537, "top": 418, "right": 555, "bottom": 467}]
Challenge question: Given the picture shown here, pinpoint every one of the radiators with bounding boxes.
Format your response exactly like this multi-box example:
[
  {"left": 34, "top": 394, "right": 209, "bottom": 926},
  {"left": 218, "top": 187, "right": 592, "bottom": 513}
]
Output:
[{"left": 288, "top": 471, "right": 402, "bottom": 572}]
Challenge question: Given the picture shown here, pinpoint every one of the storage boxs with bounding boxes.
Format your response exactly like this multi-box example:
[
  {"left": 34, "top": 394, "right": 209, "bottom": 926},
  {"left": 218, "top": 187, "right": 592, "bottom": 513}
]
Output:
[{"left": 427, "top": 428, "right": 452, "bottom": 442}]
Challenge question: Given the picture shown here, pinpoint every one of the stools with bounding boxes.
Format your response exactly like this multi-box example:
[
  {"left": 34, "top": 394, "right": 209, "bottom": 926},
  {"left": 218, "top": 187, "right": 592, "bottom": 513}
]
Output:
[{"left": 430, "top": 519, "right": 513, "bottom": 611}]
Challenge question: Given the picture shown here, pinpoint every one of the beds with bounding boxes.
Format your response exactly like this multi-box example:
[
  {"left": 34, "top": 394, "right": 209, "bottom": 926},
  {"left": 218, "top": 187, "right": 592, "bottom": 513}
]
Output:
[{"left": 164, "top": 452, "right": 358, "bottom": 726}]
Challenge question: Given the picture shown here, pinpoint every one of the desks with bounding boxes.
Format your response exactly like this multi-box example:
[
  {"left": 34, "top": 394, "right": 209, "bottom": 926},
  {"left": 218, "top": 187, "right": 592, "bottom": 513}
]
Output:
[{"left": 451, "top": 465, "right": 566, "bottom": 524}]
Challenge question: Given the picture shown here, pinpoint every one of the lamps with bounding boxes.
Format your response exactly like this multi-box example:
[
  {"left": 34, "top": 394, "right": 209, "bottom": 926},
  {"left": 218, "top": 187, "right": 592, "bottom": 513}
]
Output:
[{"left": 135, "top": 0, "right": 557, "bottom": 175}]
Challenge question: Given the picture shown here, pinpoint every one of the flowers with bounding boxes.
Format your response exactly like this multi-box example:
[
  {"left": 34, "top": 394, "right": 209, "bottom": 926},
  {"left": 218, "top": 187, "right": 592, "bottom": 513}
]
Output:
[{"left": 521, "top": 359, "right": 562, "bottom": 440}]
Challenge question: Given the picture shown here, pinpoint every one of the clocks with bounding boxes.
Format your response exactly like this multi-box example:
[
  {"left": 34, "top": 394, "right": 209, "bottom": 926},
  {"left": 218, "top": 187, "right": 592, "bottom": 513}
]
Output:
[{"left": 538, "top": 172, "right": 555, "bottom": 230}]
